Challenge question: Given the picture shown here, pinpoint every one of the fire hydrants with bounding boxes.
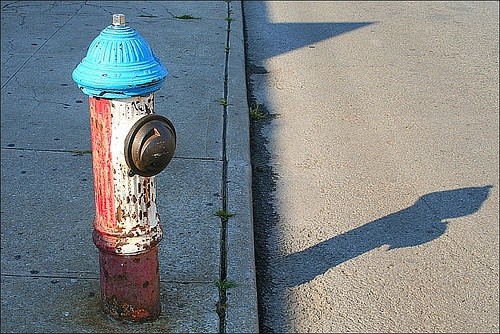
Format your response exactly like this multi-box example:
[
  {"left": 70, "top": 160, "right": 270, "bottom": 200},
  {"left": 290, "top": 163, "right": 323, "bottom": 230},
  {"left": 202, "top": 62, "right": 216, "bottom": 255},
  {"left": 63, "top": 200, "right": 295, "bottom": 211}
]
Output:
[{"left": 69, "top": 10, "right": 178, "bottom": 325}]
